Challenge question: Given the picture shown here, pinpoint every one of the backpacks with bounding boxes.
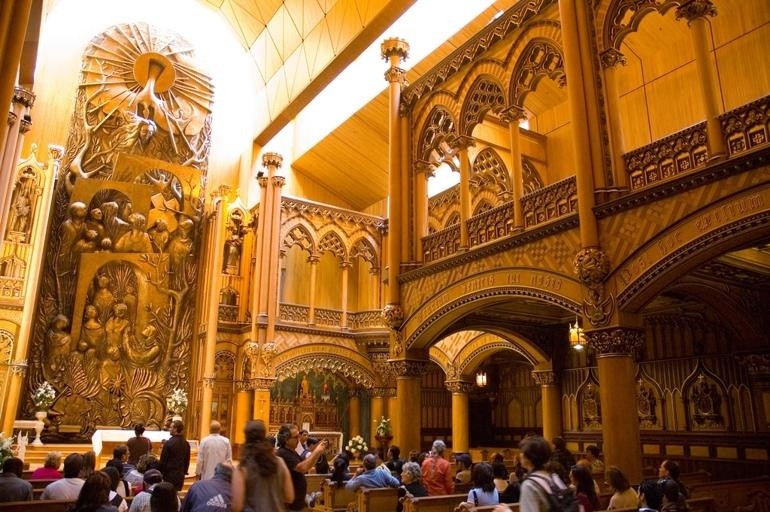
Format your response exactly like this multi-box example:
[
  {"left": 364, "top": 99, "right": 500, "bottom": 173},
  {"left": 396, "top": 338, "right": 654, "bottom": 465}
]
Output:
[{"left": 524, "top": 470, "right": 580, "bottom": 512}]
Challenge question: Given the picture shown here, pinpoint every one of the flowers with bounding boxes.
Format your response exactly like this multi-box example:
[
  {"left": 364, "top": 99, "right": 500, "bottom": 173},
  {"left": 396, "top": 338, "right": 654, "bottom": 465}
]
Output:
[
  {"left": 166, "top": 388, "right": 187, "bottom": 414},
  {"left": 31, "top": 382, "right": 55, "bottom": 412},
  {"left": 373, "top": 416, "right": 393, "bottom": 442},
  {"left": 345, "top": 435, "right": 368, "bottom": 453}
]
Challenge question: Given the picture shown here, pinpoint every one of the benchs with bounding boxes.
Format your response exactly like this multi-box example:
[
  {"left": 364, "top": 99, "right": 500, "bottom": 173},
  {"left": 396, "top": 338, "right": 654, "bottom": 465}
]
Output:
[{"left": 0, "top": 470, "right": 134, "bottom": 512}]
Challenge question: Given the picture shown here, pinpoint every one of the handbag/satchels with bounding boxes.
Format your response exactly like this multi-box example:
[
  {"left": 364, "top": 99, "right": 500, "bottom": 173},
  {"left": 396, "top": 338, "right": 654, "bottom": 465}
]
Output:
[{"left": 137, "top": 455, "right": 157, "bottom": 473}]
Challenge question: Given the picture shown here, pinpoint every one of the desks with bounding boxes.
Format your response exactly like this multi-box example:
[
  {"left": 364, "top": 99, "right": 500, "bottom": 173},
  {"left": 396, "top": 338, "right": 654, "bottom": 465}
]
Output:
[
  {"left": 92, "top": 429, "right": 171, "bottom": 470},
  {"left": 274, "top": 431, "right": 343, "bottom": 464}
]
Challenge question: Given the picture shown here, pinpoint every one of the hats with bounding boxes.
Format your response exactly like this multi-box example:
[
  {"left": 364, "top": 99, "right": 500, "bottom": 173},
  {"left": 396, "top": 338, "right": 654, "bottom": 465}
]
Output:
[
  {"left": 144, "top": 469, "right": 163, "bottom": 484},
  {"left": 456, "top": 454, "right": 472, "bottom": 468}
]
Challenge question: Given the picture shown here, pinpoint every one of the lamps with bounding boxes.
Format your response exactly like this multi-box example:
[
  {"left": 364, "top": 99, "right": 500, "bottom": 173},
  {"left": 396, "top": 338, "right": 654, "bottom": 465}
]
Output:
[
  {"left": 476, "top": 370, "right": 487, "bottom": 388},
  {"left": 568, "top": 314, "right": 586, "bottom": 350}
]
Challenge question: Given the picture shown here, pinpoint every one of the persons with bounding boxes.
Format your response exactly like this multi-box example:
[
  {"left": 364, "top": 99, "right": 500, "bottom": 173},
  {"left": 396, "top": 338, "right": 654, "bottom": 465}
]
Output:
[
  {"left": 225, "top": 225, "right": 243, "bottom": 272},
  {"left": 9, "top": 187, "right": 33, "bottom": 232},
  {"left": 1, "top": 416, "right": 691, "bottom": 512}
]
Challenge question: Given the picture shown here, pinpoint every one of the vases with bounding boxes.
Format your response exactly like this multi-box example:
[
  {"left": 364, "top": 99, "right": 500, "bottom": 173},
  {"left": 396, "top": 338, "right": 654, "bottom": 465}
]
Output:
[
  {"left": 172, "top": 414, "right": 182, "bottom": 421},
  {"left": 352, "top": 450, "right": 361, "bottom": 461},
  {"left": 32, "top": 412, "right": 47, "bottom": 445},
  {"left": 381, "top": 442, "right": 388, "bottom": 460}
]
